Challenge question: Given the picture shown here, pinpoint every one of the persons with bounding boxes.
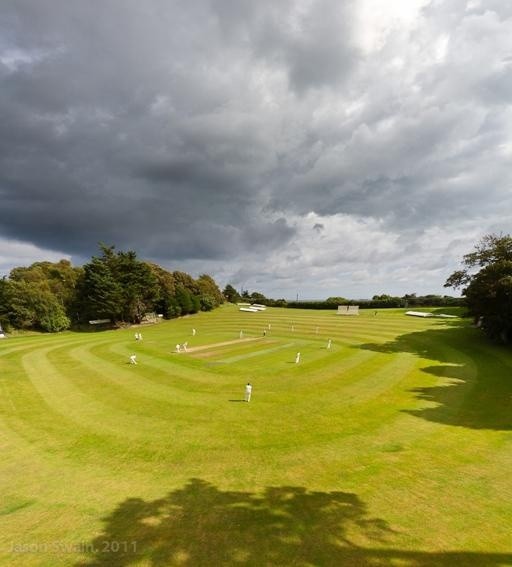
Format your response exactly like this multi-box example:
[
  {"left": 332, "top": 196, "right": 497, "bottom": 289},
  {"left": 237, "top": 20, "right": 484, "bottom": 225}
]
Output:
[
  {"left": 138, "top": 333, "right": 141, "bottom": 340},
  {"left": 327, "top": 339, "right": 331, "bottom": 349},
  {"left": 130, "top": 352, "right": 136, "bottom": 364},
  {"left": 135, "top": 332, "right": 138, "bottom": 340},
  {"left": 295, "top": 351, "right": 300, "bottom": 364},
  {"left": 263, "top": 329, "right": 266, "bottom": 336},
  {"left": 192, "top": 327, "right": 195, "bottom": 335},
  {"left": 182, "top": 342, "right": 187, "bottom": 351},
  {"left": 244, "top": 383, "right": 253, "bottom": 402},
  {"left": 176, "top": 343, "right": 181, "bottom": 352},
  {"left": 240, "top": 330, "right": 242, "bottom": 338}
]
